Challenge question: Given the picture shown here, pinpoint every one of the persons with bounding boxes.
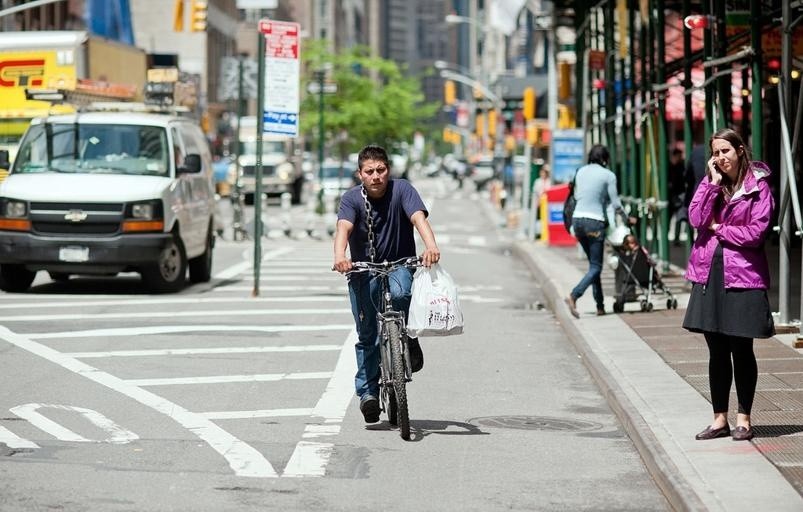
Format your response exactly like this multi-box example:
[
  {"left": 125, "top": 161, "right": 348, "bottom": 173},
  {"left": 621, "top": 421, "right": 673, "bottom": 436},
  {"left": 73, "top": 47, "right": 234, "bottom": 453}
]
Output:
[
  {"left": 333, "top": 147, "right": 440, "bottom": 422},
  {"left": 531, "top": 165, "right": 551, "bottom": 241},
  {"left": 624, "top": 234, "right": 638, "bottom": 252},
  {"left": 667, "top": 148, "right": 695, "bottom": 233},
  {"left": 564, "top": 144, "right": 638, "bottom": 319},
  {"left": 682, "top": 128, "right": 776, "bottom": 441}
]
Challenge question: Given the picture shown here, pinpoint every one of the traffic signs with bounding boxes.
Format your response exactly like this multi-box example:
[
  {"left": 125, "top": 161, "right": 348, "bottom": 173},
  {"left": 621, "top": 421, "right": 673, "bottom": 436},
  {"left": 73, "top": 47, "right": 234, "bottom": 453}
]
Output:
[{"left": 304, "top": 82, "right": 339, "bottom": 100}]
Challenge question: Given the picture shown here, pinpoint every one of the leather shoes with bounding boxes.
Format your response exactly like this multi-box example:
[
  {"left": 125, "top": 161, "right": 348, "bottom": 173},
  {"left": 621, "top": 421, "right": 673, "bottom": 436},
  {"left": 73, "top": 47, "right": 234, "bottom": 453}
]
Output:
[
  {"left": 695, "top": 421, "right": 731, "bottom": 441},
  {"left": 564, "top": 296, "right": 579, "bottom": 319},
  {"left": 733, "top": 417, "right": 754, "bottom": 440},
  {"left": 596, "top": 307, "right": 607, "bottom": 315}
]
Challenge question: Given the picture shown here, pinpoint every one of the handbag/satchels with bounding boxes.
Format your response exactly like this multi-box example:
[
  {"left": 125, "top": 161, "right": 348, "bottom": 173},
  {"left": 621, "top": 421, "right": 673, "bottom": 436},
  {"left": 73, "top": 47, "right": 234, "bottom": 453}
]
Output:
[{"left": 564, "top": 195, "right": 577, "bottom": 235}]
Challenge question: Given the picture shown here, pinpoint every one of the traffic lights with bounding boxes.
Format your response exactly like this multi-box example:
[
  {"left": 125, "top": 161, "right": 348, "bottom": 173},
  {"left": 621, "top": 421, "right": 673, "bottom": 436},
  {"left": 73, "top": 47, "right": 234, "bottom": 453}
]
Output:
[
  {"left": 190, "top": 0, "right": 210, "bottom": 34},
  {"left": 521, "top": 86, "right": 534, "bottom": 124},
  {"left": 554, "top": 60, "right": 571, "bottom": 101},
  {"left": 530, "top": 124, "right": 552, "bottom": 149}
]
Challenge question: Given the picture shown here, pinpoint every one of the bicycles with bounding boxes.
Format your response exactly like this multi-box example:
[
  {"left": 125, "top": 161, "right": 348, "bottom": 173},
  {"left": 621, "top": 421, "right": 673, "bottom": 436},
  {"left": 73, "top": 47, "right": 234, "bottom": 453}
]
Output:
[{"left": 331, "top": 257, "right": 435, "bottom": 439}]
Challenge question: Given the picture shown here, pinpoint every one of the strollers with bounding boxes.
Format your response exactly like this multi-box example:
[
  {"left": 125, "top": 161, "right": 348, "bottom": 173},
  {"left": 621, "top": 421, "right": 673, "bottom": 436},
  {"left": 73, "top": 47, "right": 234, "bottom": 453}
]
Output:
[{"left": 606, "top": 218, "right": 680, "bottom": 316}]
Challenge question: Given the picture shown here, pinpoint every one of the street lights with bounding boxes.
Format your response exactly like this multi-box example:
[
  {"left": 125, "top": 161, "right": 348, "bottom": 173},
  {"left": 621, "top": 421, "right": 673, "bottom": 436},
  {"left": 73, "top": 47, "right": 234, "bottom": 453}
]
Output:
[
  {"left": 230, "top": 50, "right": 249, "bottom": 157},
  {"left": 426, "top": 0, "right": 518, "bottom": 123}
]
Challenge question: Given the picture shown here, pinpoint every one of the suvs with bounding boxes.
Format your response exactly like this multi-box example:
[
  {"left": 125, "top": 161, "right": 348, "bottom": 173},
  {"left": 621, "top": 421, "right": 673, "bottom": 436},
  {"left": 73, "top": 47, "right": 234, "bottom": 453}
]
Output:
[
  {"left": 226, "top": 131, "right": 301, "bottom": 210},
  {"left": 0, "top": 114, "right": 217, "bottom": 290}
]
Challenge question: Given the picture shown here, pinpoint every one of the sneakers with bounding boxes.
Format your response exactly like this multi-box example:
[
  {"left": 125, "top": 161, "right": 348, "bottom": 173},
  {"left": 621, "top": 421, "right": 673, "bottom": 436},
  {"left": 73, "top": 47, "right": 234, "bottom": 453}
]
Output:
[
  {"left": 406, "top": 334, "right": 424, "bottom": 373},
  {"left": 359, "top": 396, "right": 381, "bottom": 424}
]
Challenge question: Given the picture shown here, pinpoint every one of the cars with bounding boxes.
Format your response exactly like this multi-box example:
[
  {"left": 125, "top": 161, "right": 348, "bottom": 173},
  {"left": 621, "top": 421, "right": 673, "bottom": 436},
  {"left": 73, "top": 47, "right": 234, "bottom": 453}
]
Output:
[
  {"left": 388, "top": 148, "right": 541, "bottom": 195},
  {"left": 307, "top": 164, "right": 354, "bottom": 217}
]
